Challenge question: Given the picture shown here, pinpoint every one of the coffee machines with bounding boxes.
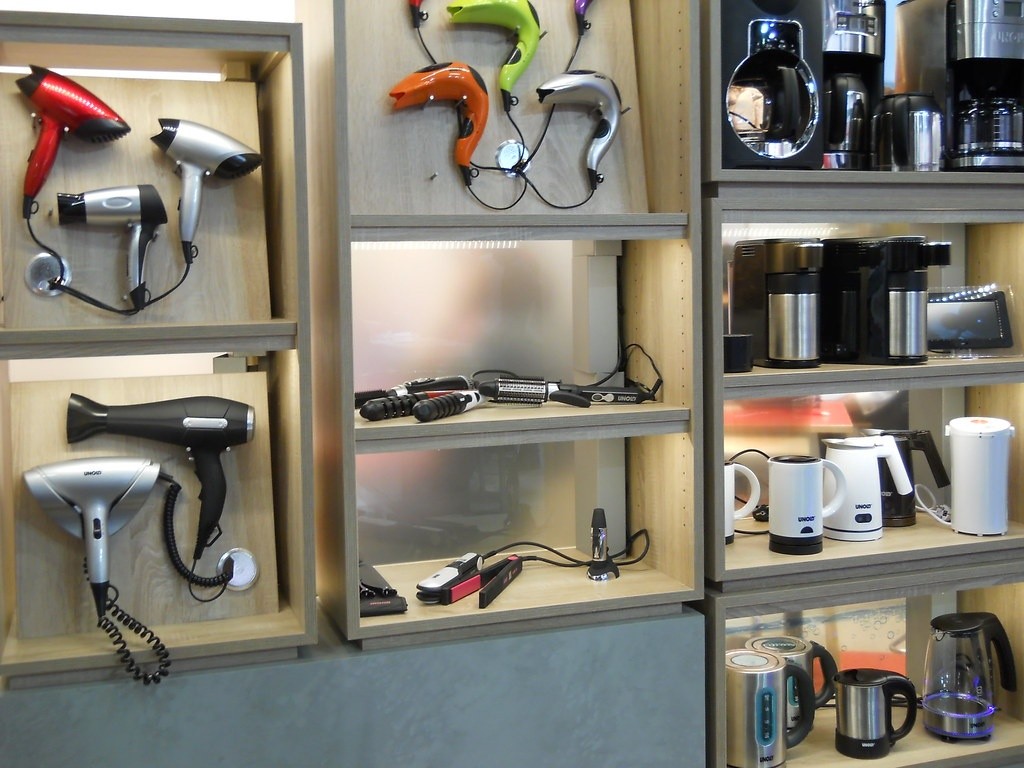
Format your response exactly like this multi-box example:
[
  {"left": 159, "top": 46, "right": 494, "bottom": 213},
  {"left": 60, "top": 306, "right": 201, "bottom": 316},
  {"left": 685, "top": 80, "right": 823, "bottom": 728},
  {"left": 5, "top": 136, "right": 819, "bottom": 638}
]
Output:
[
  {"left": 729, "top": 238, "right": 825, "bottom": 369},
  {"left": 818, "top": 236, "right": 952, "bottom": 365},
  {"left": 892, "top": 0, "right": 1024, "bottom": 172}
]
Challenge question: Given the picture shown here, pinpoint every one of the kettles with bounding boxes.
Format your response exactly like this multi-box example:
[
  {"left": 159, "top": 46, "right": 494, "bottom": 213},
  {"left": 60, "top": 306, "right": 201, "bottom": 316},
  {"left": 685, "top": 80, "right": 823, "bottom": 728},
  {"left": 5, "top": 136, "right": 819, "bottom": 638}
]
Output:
[
  {"left": 825, "top": 71, "right": 870, "bottom": 151},
  {"left": 831, "top": 668, "right": 918, "bottom": 760},
  {"left": 945, "top": 416, "right": 1015, "bottom": 537},
  {"left": 820, "top": 436, "right": 913, "bottom": 541},
  {"left": 921, "top": 612, "right": 1018, "bottom": 744},
  {"left": 872, "top": 93, "right": 946, "bottom": 173},
  {"left": 766, "top": 454, "right": 847, "bottom": 556},
  {"left": 859, "top": 427, "right": 951, "bottom": 528},
  {"left": 725, "top": 460, "right": 761, "bottom": 546}
]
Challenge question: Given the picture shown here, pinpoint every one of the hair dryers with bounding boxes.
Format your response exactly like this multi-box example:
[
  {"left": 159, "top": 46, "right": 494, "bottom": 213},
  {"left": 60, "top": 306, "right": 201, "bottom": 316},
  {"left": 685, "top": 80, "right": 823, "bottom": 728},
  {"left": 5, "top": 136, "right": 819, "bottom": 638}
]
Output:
[
  {"left": 389, "top": 60, "right": 489, "bottom": 187},
  {"left": 56, "top": 183, "right": 169, "bottom": 311},
  {"left": 65, "top": 391, "right": 257, "bottom": 561},
  {"left": 537, "top": 69, "right": 622, "bottom": 192},
  {"left": 150, "top": 117, "right": 262, "bottom": 265},
  {"left": 445, "top": 0, "right": 540, "bottom": 111},
  {"left": 20, "top": 456, "right": 161, "bottom": 616},
  {"left": 14, "top": 62, "right": 132, "bottom": 219}
]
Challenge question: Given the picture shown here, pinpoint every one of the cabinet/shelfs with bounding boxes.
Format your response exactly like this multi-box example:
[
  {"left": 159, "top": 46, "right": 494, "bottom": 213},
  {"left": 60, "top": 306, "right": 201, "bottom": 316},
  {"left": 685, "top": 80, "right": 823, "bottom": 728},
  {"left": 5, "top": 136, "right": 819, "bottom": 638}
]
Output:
[
  {"left": 700, "top": 1, "right": 1024, "bottom": 767},
  {"left": 1, "top": 11, "right": 320, "bottom": 690},
  {"left": 295, "top": 0, "right": 706, "bottom": 653}
]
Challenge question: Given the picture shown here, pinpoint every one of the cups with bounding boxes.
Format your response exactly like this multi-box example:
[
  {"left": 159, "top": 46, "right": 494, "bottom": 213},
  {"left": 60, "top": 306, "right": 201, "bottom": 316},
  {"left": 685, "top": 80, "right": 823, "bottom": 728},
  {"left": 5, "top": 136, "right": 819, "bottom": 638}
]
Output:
[{"left": 723, "top": 334, "right": 755, "bottom": 373}]
